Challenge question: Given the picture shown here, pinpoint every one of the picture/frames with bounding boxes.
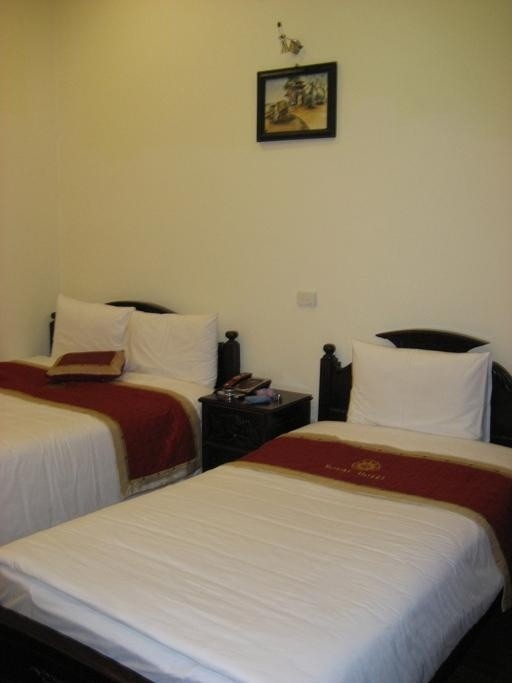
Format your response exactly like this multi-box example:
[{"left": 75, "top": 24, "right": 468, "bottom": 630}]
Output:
[{"left": 256, "top": 61, "right": 337, "bottom": 143}]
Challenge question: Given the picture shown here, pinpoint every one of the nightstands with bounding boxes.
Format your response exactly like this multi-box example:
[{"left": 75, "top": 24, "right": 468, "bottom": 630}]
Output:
[{"left": 198, "top": 387, "right": 313, "bottom": 474}]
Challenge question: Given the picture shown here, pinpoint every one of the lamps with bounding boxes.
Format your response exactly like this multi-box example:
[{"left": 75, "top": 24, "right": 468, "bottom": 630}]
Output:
[{"left": 276, "top": 21, "right": 303, "bottom": 55}]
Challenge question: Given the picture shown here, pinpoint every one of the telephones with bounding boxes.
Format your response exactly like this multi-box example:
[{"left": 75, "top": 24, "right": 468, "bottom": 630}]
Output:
[{"left": 224, "top": 372, "right": 272, "bottom": 394}]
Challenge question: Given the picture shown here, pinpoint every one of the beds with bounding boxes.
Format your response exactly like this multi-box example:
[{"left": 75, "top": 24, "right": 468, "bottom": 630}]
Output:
[
  {"left": 0, "top": 328, "right": 512, "bottom": 683},
  {"left": 0, "top": 301, "right": 241, "bottom": 551}
]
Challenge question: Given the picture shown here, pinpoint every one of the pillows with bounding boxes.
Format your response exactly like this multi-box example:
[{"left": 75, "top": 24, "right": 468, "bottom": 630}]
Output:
[
  {"left": 50, "top": 292, "right": 134, "bottom": 370},
  {"left": 123, "top": 310, "right": 220, "bottom": 389},
  {"left": 346, "top": 330, "right": 491, "bottom": 442},
  {"left": 46, "top": 349, "right": 125, "bottom": 382}
]
